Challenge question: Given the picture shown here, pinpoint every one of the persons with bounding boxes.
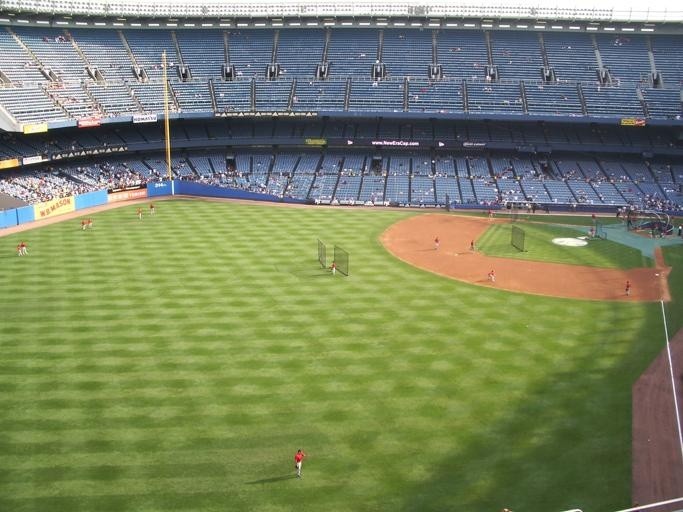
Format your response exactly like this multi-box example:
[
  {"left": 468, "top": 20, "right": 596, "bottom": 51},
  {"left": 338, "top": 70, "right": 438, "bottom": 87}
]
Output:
[
  {"left": 678, "top": 224, "right": 682, "bottom": 236},
  {"left": 435, "top": 236, "right": 440, "bottom": 248},
  {"left": 623, "top": 280, "right": 632, "bottom": 296},
  {"left": 488, "top": 210, "right": 492, "bottom": 219},
  {"left": 0, "top": 29, "right": 683, "bottom": 214},
  {"left": 295, "top": 449, "right": 305, "bottom": 479},
  {"left": 616, "top": 204, "right": 634, "bottom": 228},
  {"left": 590, "top": 227, "right": 595, "bottom": 237},
  {"left": 17, "top": 242, "right": 28, "bottom": 256},
  {"left": 651, "top": 224, "right": 664, "bottom": 238},
  {"left": 81, "top": 218, "right": 92, "bottom": 230},
  {"left": 330, "top": 261, "right": 335, "bottom": 275},
  {"left": 488, "top": 269, "right": 495, "bottom": 282},
  {"left": 138, "top": 208, "right": 142, "bottom": 219},
  {"left": 470, "top": 240, "right": 474, "bottom": 250}
]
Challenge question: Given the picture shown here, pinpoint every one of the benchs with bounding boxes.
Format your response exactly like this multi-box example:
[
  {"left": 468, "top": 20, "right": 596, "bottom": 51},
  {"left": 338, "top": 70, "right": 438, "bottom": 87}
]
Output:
[{"left": 0, "top": 29, "right": 682, "bottom": 225}]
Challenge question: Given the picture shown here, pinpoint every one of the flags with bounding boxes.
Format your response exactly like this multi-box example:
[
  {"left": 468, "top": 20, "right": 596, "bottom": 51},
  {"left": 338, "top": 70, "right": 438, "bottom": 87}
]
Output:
[{"left": 592, "top": 214, "right": 596, "bottom": 219}]
